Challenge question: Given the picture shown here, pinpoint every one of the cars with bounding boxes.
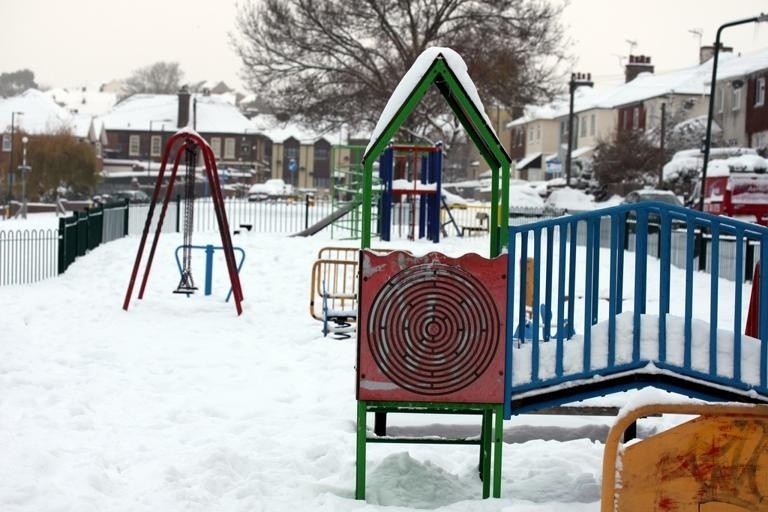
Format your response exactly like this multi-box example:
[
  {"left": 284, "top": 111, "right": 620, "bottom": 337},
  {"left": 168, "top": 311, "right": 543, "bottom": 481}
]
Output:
[{"left": 510, "top": 154, "right": 768, "bottom": 237}]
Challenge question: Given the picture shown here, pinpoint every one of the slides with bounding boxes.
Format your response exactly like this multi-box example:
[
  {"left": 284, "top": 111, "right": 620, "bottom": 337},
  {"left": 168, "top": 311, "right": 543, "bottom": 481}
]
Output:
[{"left": 288, "top": 200, "right": 362, "bottom": 237}]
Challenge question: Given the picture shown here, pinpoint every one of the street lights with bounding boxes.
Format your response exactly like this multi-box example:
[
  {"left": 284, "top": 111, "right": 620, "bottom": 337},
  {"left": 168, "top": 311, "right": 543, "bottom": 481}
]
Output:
[
  {"left": 6, "top": 110, "right": 24, "bottom": 217},
  {"left": 697, "top": 14, "right": 768, "bottom": 213},
  {"left": 568, "top": 78, "right": 593, "bottom": 187},
  {"left": 20, "top": 135, "right": 29, "bottom": 219}
]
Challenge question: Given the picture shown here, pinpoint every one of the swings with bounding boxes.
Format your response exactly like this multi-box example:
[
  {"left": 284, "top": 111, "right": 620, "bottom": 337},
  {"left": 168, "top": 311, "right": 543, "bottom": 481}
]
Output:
[{"left": 172, "top": 142, "right": 199, "bottom": 294}]
